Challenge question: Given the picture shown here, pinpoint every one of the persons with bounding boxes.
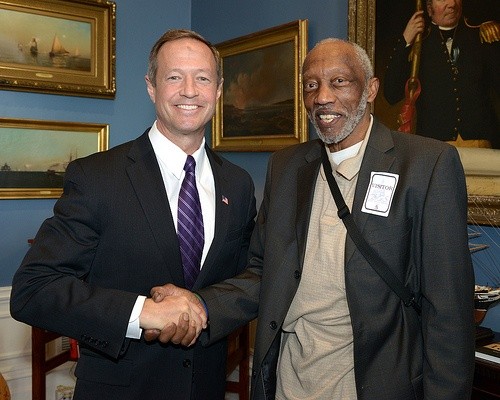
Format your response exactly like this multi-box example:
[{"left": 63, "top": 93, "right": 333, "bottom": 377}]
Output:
[
  {"left": 10, "top": 30, "right": 254, "bottom": 400},
  {"left": 145, "top": 38, "right": 478, "bottom": 400}
]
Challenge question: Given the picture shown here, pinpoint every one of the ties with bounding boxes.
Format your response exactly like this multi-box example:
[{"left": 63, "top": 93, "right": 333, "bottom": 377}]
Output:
[{"left": 178, "top": 156, "right": 206, "bottom": 282}]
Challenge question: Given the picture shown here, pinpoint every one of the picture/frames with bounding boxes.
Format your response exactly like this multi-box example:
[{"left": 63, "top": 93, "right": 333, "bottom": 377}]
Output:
[
  {"left": 348, "top": 0, "right": 499, "bottom": 228},
  {"left": 210, "top": 17, "right": 308, "bottom": 152},
  {"left": 0, "top": 117, "right": 110, "bottom": 199},
  {"left": 0, "top": 0, "right": 117, "bottom": 100}
]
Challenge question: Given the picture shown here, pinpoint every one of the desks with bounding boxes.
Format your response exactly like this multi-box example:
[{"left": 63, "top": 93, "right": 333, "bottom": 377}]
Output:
[{"left": 472, "top": 326, "right": 500, "bottom": 400}]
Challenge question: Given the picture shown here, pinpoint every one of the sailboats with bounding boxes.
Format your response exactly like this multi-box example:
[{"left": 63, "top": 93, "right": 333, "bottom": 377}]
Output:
[{"left": 467, "top": 193, "right": 500, "bottom": 324}]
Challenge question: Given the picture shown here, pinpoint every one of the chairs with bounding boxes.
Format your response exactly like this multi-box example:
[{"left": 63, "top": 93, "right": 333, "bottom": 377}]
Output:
[
  {"left": 224, "top": 324, "right": 251, "bottom": 400},
  {"left": 31, "top": 324, "right": 70, "bottom": 400}
]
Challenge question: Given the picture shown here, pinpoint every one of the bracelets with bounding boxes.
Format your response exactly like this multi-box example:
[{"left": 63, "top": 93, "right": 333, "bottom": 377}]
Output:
[{"left": 194, "top": 293, "right": 209, "bottom": 315}]
